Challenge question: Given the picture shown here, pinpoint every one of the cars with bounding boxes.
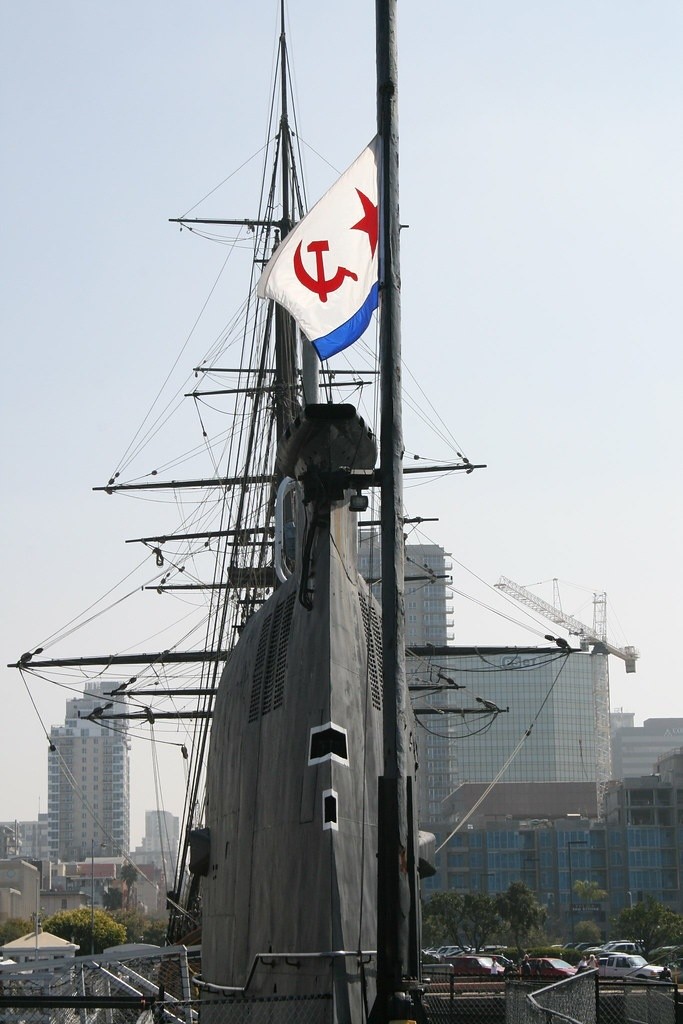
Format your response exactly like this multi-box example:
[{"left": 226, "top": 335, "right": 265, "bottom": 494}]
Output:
[{"left": 421, "top": 938, "right": 683, "bottom": 984}]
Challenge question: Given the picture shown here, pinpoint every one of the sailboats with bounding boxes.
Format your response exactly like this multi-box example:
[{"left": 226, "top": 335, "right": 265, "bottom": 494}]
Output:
[{"left": 4, "top": 1, "right": 582, "bottom": 1024}]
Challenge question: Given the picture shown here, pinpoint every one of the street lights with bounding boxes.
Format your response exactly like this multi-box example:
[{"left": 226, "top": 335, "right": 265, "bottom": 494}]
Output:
[{"left": 568, "top": 841, "right": 588, "bottom": 951}]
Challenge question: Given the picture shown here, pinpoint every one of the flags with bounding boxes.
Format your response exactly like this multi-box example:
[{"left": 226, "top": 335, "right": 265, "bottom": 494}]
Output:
[{"left": 259, "top": 139, "right": 381, "bottom": 362}]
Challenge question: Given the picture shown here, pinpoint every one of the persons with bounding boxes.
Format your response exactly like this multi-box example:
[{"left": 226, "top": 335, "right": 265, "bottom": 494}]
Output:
[{"left": 491, "top": 951, "right": 672, "bottom": 992}]
